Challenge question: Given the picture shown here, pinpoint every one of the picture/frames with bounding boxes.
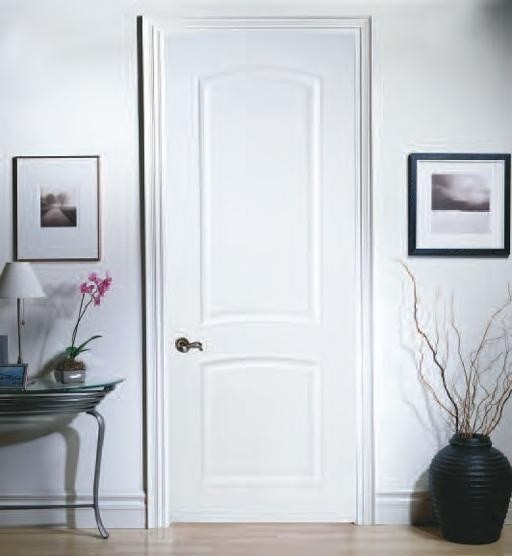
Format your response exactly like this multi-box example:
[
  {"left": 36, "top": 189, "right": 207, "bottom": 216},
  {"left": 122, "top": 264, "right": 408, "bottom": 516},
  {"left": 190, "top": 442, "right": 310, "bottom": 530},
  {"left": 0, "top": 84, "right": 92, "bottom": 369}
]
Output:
[
  {"left": 12, "top": 155, "right": 102, "bottom": 261},
  {"left": 407, "top": 153, "right": 512, "bottom": 257}
]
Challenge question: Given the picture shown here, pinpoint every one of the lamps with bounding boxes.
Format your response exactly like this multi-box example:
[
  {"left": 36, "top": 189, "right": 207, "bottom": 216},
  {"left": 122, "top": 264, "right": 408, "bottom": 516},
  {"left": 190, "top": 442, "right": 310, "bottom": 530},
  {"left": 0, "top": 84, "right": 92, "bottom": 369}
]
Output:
[{"left": 0, "top": 262, "right": 48, "bottom": 364}]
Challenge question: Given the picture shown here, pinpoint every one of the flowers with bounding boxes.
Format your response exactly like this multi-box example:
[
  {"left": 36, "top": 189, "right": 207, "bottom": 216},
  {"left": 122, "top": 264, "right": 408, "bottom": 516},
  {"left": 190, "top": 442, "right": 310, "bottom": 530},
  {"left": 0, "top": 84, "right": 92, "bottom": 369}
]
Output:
[{"left": 65, "top": 269, "right": 114, "bottom": 363}]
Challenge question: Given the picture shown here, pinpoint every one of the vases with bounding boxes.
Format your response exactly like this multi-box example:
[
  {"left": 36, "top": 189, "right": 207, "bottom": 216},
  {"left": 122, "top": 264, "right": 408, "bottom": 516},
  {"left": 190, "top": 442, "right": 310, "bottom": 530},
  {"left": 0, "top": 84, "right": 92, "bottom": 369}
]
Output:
[
  {"left": 54, "top": 363, "right": 87, "bottom": 386},
  {"left": 426, "top": 430, "right": 512, "bottom": 547}
]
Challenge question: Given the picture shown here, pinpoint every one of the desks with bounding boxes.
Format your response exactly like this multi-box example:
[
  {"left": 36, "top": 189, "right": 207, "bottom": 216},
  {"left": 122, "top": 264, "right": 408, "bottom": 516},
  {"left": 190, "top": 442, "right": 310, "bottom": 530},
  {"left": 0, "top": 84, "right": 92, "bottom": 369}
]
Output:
[{"left": 1, "top": 378, "right": 123, "bottom": 539}]
